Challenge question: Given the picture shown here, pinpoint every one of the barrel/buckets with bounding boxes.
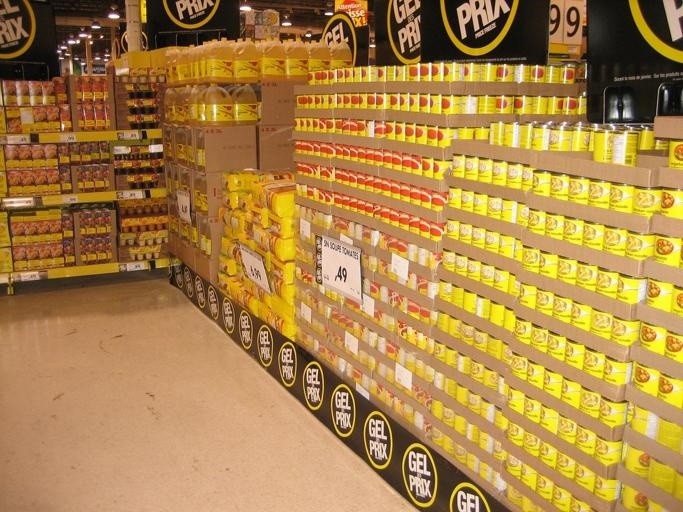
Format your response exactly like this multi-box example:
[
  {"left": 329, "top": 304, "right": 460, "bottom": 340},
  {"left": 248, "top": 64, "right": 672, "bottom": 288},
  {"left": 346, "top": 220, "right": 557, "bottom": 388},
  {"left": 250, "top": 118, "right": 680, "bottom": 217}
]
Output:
[
  {"left": 217, "top": 167, "right": 295, "bottom": 340},
  {"left": 161, "top": 36, "right": 352, "bottom": 128}
]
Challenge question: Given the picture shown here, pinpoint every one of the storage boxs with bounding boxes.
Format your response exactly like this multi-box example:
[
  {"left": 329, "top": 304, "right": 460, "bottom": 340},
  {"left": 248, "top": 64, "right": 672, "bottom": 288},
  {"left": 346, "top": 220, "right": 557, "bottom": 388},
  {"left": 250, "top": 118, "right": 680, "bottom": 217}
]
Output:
[{"left": 168, "top": 79, "right": 306, "bottom": 283}]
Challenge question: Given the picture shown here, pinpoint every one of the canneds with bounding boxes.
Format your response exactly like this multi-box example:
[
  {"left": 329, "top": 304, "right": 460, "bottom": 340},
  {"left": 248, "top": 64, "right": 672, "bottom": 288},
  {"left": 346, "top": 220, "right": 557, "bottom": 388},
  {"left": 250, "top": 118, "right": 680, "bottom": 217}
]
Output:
[{"left": 290, "top": 62, "right": 683, "bottom": 512}]
